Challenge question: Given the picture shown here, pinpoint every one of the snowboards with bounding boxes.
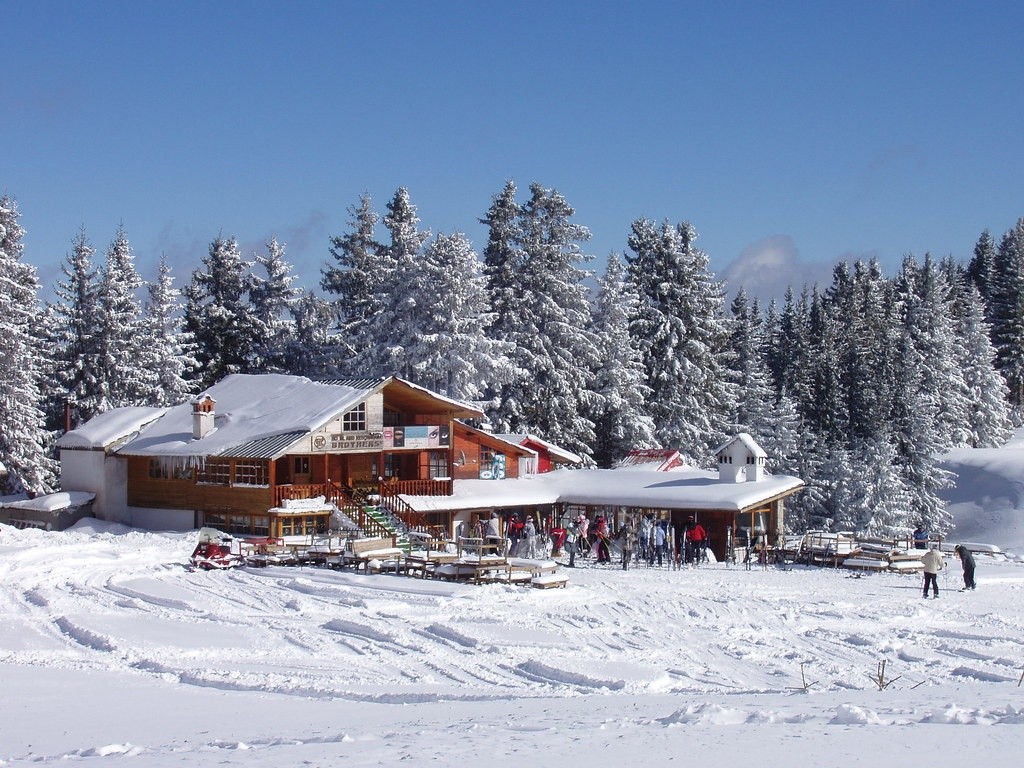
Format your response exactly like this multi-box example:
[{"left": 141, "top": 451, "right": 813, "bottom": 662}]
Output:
[{"left": 958, "top": 582, "right": 977, "bottom": 593}]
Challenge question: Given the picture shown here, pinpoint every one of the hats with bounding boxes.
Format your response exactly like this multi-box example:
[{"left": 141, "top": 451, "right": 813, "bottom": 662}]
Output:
[
  {"left": 527, "top": 516, "right": 534, "bottom": 522},
  {"left": 954, "top": 545, "right": 960, "bottom": 551},
  {"left": 932, "top": 544, "right": 937, "bottom": 549},
  {"left": 491, "top": 513, "right": 497, "bottom": 517},
  {"left": 511, "top": 513, "right": 518, "bottom": 517},
  {"left": 918, "top": 524, "right": 924, "bottom": 530}
]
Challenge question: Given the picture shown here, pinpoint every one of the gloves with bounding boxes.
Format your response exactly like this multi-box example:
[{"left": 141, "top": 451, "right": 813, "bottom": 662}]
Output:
[{"left": 945, "top": 562, "right": 947, "bottom": 566}]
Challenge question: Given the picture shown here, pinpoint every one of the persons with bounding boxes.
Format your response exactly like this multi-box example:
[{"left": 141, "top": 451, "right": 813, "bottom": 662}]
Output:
[
  {"left": 641, "top": 513, "right": 665, "bottom": 566},
  {"left": 912, "top": 525, "right": 927, "bottom": 549},
  {"left": 622, "top": 518, "right": 638, "bottom": 571},
  {"left": 563, "top": 514, "right": 611, "bottom": 566},
  {"left": 955, "top": 545, "right": 976, "bottom": 590},
  {"left": 920, "top": 545, "right": 943, "bottom": 599},
  {"left": 686, "top": 515, "right": 707, "bottom": 564},
  {"left": 487, "top": 512, "right": 536, "bottom": 559}
]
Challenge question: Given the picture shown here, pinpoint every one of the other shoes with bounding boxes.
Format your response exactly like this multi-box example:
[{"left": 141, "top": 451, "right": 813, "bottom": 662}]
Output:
[
  {"left": 597, "top": 558, "right": 605, "bottom": 562},
  {"left": 551, "top": 553, "right": 561, "bottom": 557},
  {"left": 569, "top": 563, "right": 575, "bottom": 567},
  {"left": 923, "top": 593, "right": 929, "bottom": 597},
  {"left": 658, "top": 562, "right": 662, "bottom": 566},
  {"left": 934, "top": 594, "right": 940, "bottom": 598},
  {"left": 962, "top": 586, "right": 971, "bottom": 590},
  {"left": 604, "top": 558, "right": 611, "bottom": 562},
  {"left": 650, "top": 561, "right": 654, "bottom": 565},
  {"left": 972, "top": 582, "right": 976, "bottom": 589}
]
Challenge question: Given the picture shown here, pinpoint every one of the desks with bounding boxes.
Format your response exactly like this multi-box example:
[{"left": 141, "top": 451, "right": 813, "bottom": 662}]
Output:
[{"left": 350, "top": 532, "right": 561, "bottom": 586}]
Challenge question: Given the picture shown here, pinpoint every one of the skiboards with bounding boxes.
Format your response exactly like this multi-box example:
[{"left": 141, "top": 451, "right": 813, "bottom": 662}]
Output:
[{"left": 553, "top": 560, "right": 583, "bottom": 569}]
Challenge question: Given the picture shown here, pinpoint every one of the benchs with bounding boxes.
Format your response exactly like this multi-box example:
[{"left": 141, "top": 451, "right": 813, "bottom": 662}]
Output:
[{"left": 533, "top": 575, "right": 571, "bottom": 588}]
